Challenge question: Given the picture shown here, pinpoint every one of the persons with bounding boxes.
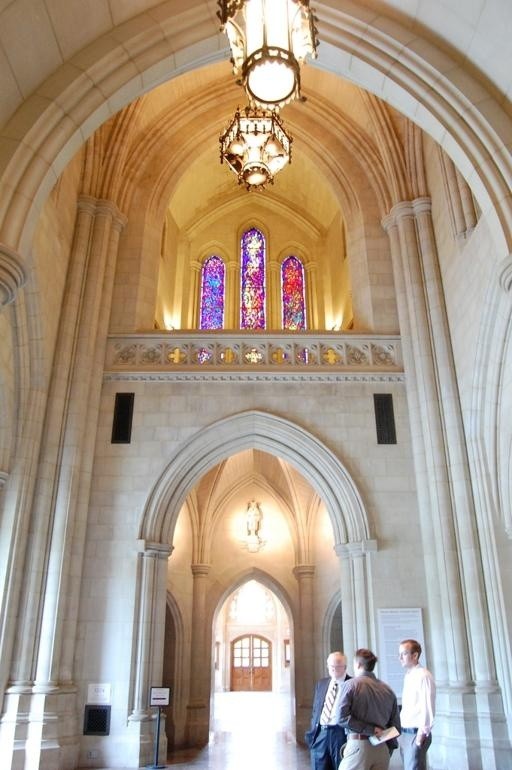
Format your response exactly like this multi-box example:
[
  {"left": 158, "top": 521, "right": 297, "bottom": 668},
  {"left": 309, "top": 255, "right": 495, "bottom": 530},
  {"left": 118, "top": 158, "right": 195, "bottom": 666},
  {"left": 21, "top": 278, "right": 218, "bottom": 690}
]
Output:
[{"left": 304, "top": 639, "right": 436, "bottom": 770}]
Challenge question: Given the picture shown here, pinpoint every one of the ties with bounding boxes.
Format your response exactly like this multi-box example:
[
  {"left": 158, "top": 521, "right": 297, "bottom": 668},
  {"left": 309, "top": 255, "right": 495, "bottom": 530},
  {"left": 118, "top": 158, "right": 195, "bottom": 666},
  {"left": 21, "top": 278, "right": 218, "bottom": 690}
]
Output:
[{"left": 320, "top": 681, "right": 337, "bottom": 724}]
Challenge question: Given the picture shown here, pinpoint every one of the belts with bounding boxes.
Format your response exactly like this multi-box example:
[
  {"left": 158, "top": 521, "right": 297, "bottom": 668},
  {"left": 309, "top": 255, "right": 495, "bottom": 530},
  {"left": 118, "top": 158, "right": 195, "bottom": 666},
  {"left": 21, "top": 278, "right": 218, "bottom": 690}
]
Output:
[
  {"left": 400, "top": 728, "right": 418, "bottom": 734},
  {"left": 347, "top": 734, "right": 369, "bottom": 740}
]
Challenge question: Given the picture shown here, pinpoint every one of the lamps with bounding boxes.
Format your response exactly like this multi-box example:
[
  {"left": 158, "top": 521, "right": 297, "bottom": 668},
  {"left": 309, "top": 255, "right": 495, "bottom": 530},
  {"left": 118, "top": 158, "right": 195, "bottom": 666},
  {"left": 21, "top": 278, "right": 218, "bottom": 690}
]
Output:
[
  {"left": 218, "top": 103, "right": 294, "bottom": 195},
  {"left": 211, "top": 1, "right": 320, "bottom": 114}
]
